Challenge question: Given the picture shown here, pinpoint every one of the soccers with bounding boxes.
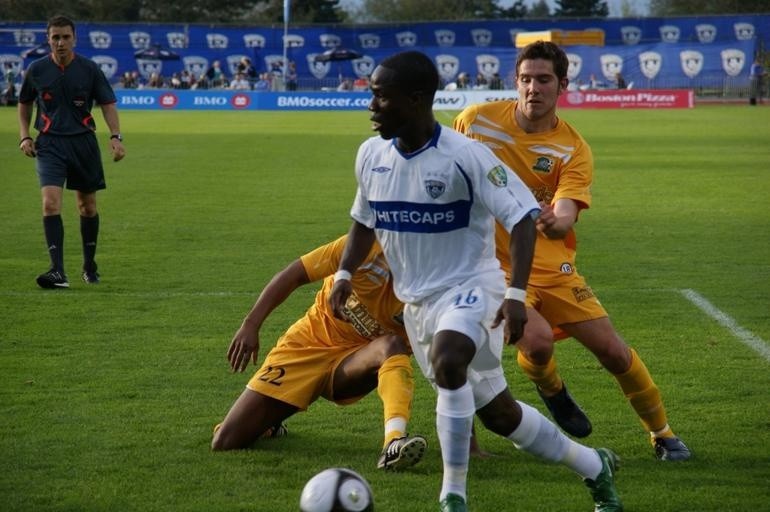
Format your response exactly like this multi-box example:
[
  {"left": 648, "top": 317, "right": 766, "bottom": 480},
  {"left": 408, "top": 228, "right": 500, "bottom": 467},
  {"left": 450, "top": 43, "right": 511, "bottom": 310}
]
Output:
[{"left": 299, "top": 468, "right": 375, "bottom": 511}]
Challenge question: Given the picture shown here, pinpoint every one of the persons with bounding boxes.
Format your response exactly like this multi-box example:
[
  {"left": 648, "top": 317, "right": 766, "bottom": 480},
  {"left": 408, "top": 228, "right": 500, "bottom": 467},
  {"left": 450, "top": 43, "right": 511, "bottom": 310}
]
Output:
[
  {"left": 451, "top": 41, "right": 693, "bottom": 464},
  {"left": 747, "top": 57, "right": 769, "bottom": 105},
  {"left": 616, "top": 72, "right": 627, "bottom": 89},
  {"left": 327, "top": 50, "right": 623, "bottom": 511},
  {"left": 122, "top": 58, "right": 298, "bottom": 92},
  {"left": 589, "top": 73, "right": 598, "bottom": 88},
  {"left": 448, "top": 72, "right": 504, "bottom": 90},
  {"left": 208, "top": 230, "right": 501, "bottom": 468},
  {"left": 3, "top": 69, "right": 15, "bottom": 108},
  {"left": 15, "top": 15, "right": 129, "bottom": 290},
  {"left": 339, "top": 76, "right": 370, "bottom": 91}
]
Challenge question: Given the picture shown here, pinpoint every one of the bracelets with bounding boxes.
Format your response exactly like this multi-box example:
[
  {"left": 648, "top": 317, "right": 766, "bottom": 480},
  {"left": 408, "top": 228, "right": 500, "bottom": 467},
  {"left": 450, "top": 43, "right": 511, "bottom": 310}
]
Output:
[
  {"left": 108, "top": 134, "right": 123, "bottom": 141},
  {"left": 331, "top": 270, "right": 352, "bottom": 283},
  {"left": 503, "top": 285, "right": 528, "bottom": 306},
  {"left": 18, "top": 137, "right": 33, "bottom": 147}
]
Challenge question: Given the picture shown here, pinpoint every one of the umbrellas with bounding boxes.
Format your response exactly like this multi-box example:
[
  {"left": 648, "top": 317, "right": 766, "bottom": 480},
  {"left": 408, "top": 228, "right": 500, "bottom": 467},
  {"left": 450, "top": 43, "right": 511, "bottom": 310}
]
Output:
[
  {"left": 313, "top": 47, "right": 364, "bottom": 79},
  {"left": 133, "top": 42, "right": 182, "bottom": 73},
  {"left": 19, "top": 40, "right": 52, "bottom": 61}
]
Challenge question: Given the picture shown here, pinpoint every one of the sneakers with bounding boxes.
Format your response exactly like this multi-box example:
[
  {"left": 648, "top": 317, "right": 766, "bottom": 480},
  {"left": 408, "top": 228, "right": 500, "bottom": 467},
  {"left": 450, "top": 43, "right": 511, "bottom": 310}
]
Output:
[
  {"left": 654, "top": 437, "right": 692, "bottom": 462},
  {"left": 440, "top": 491, "right": 469, "bottom": 512},
  {"left": 581, "top": 446, "right": 623, "bottom": 512},
  {"left": 80, "top": 269, "right": 98, "bottom": 284},
  {"left": 271, "top": 419, "right": 288, "bottom": 441},
  {"left": 35, "top": 269, "right": 69, "bottom": 288},
  {"left": 376, "top": 434, "right": 427, "bottom": 471},
  {"left": 537, "top": 382, "right": 593, "bottom": 437}
]
